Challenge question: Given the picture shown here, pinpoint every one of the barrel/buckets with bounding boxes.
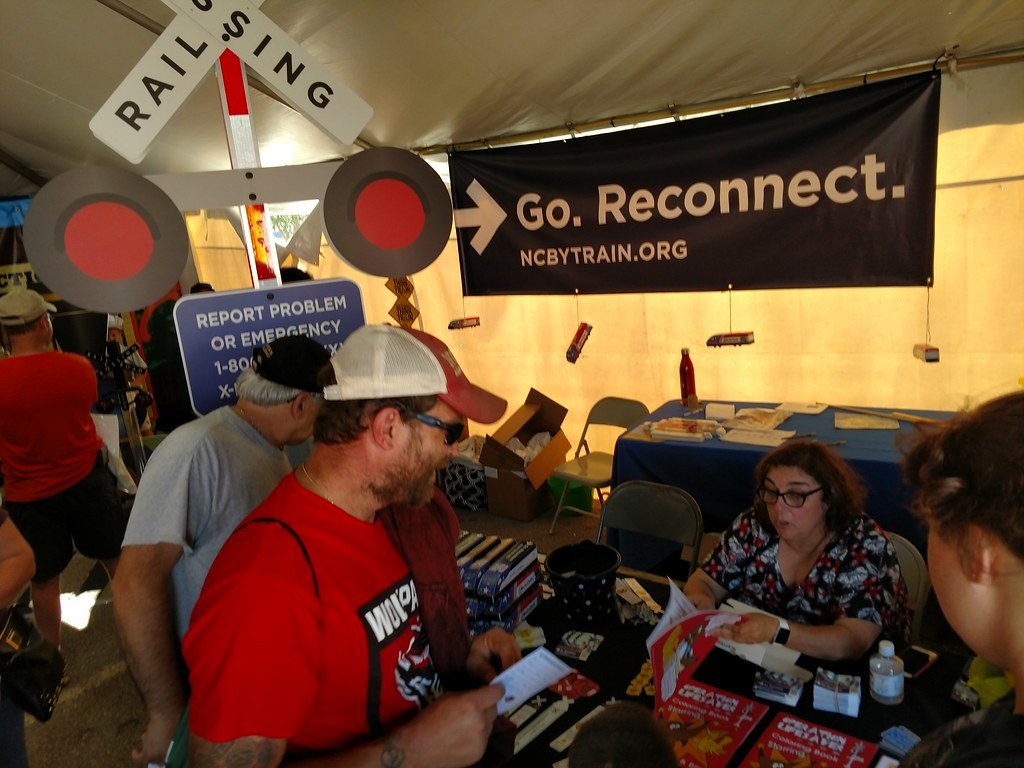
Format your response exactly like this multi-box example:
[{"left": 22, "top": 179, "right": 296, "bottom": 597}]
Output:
[
  {"left": 544, "top": 543, "right": 622, "bottom": 628},
  {"left": 548, "top": 471, "right": 592, "bottom": 514}
]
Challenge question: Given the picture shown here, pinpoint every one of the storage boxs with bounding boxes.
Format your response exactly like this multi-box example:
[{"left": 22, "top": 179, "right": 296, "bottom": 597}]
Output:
[{"left": 445, "top": 387, "right": 573, "bottom": 522}]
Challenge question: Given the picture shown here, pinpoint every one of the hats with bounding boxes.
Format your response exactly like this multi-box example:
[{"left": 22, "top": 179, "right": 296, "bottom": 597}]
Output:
[
  {"left": 0, "top": 287, "right": 57, "bottom": 326},
  {"left": 251, "top": 335, "right": 333, "bottom": 392},
  {"left": 322, "top": 322, "right": 508, "bottom": 423}
]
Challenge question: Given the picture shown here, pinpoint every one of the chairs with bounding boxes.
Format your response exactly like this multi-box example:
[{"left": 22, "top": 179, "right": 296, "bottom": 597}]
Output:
[
  {"left": 550, "top": 396, "right": 652, "bottom": 536},
  {"left": 883, "top": 531, "right": 930, "bottom": 646},
  {"left": 598, "top": 479, "right": 705, "bottom": 580}
]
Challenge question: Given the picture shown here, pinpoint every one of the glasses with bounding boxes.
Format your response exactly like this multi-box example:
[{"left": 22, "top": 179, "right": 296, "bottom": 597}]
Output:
[
  {"left": 371, "top": 402, "right": 465, "bottom": 445},
  {"left": 755, "top": 483, "right": 829, "bottom": 509}
]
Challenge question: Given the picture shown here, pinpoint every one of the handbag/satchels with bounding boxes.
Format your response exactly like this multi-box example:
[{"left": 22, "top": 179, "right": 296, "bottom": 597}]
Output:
[{"left": 0, "top": 637, "right": 67, "bottom": 723}]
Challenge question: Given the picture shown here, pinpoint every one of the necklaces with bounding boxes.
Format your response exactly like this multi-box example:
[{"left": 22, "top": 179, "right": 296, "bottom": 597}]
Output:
[
  {"left": 235, "top": 405, "right": 283, "bottom": 451},
  {"left": 302, "top": 462, "right": 335, "bottom": 505}
]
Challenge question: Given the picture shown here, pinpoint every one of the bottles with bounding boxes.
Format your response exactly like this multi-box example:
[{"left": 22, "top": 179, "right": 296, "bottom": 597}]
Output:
[
  {"left": 680, "top": 348, "right": 696, "bottom": 406},
  {"left": 869, "top": 640, "right": 904, "bottom": 704}
]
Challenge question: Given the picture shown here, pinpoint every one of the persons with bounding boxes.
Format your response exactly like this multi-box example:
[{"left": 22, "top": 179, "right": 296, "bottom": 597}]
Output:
[
  {"left": 684, "top": 441, "right": 912, "bottom": 662},
  {"left": 569, "top": 704, "right": 681, "bottom": 768},
  {"left": 111, "top": 335, "right": 332, "bottom": 768},
  {"left": 0, "top": 287, "right": 128, "bottom": 688},
  {"left": 182, "top": 322, "right": 523, "bottom": 768},
  {"left": 897, "top": 391, "right": 1024, "bottom": 768},
  {"left": 191, "top": 283, "right": 215, "bottom": 294},
  {"left": 0, "top": 501, "right": 36, "bottom": 768}
]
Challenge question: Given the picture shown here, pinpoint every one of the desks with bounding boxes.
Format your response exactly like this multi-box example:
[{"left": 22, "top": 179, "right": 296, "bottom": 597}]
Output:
[
  {"left": 607, "top": 399, "right": 954, "bottom": 571},
  {"left": 469, "top": 560, "right": 978, "bottom": 768}
]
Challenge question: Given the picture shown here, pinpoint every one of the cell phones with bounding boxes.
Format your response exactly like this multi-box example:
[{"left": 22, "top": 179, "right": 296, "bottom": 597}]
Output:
[{"left": 896, "top": 645, "right": 938, "bottom": 678}]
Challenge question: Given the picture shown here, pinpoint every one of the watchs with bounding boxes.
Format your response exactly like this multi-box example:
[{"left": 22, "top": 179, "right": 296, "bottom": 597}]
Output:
[{"left": 771, "top": 619, "right": 791, "bottom": 647}]
